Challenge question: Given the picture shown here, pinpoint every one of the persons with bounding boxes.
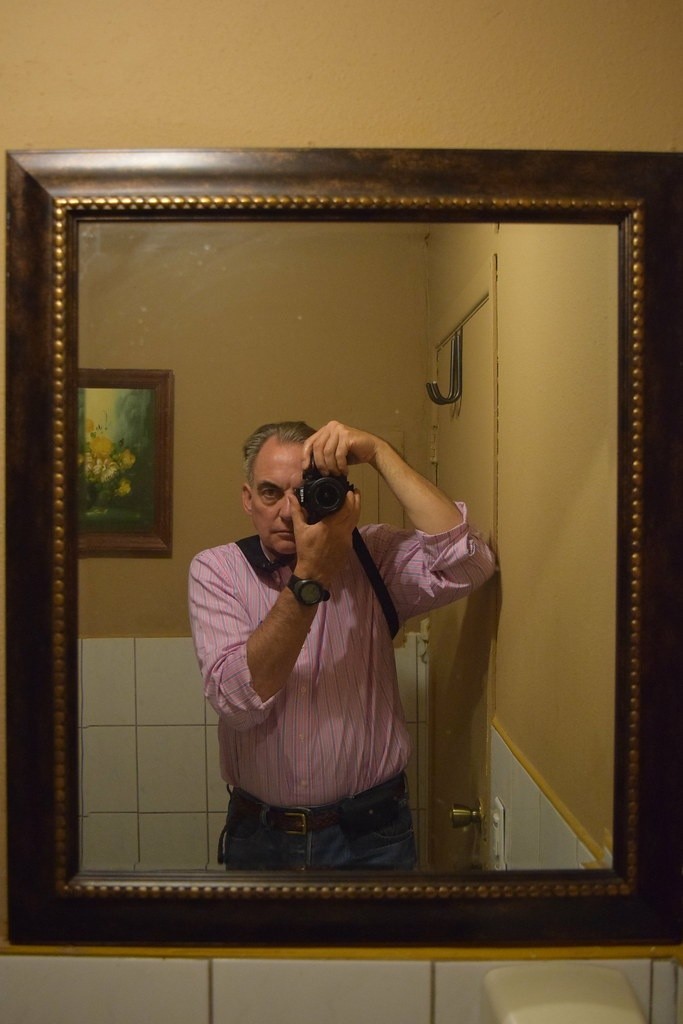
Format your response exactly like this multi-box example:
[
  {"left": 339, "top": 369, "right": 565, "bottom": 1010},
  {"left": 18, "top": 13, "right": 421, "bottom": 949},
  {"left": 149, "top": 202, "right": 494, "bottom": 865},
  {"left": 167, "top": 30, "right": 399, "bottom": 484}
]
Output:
[{"left": 188, "top": 420, "right": 497, "bottom": 872}]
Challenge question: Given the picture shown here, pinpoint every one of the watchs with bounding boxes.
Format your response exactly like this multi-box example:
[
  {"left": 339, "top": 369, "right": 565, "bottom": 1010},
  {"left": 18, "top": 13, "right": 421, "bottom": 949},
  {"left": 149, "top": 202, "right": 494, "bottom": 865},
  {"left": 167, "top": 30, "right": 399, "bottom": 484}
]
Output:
[{"left": 290, "top": 575, "right": 330, "bottom": 605}]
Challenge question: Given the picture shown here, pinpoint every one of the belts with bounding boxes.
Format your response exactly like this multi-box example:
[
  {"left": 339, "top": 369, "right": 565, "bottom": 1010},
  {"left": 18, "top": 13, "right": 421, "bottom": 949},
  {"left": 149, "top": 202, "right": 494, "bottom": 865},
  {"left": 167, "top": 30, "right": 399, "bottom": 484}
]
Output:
[{"left": 230, "top": 781, "right": 406, "bottom": 834}]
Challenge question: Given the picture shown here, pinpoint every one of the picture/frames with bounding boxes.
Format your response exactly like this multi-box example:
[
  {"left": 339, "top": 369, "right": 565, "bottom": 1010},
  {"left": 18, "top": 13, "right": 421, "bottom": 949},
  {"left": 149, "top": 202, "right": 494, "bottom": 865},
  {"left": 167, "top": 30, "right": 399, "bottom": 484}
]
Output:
[{"left": 77, "top": 368, "right": 174, "bottom": 560}]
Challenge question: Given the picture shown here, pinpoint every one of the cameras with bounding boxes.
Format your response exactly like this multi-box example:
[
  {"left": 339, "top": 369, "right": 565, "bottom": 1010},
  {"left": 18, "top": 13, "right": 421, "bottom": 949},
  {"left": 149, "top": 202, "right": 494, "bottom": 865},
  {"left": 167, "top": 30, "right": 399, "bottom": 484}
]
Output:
[{"left": 295, "top": 465, "right": 352, "bottom": 525}]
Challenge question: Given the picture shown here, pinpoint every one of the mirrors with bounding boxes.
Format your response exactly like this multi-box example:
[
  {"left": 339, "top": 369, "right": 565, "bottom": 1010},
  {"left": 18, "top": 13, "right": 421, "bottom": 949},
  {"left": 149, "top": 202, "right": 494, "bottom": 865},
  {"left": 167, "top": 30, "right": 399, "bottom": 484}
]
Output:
[{"left": 0, "top": 146, "right": 683, "bottom": 947}]
